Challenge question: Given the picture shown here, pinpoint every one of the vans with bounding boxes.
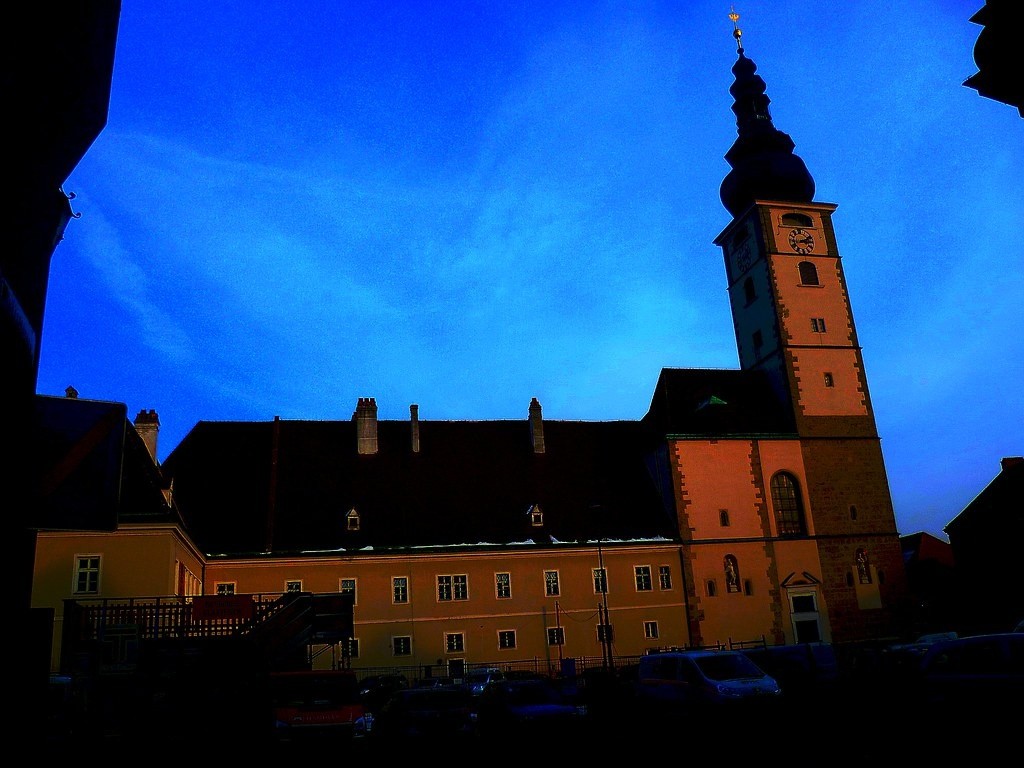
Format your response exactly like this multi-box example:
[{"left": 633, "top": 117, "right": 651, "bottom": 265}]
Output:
[
  {"left": 637, "top": 650, "right": 782, "bottom": 709},
  {"left": 462, "top": 667, "right": 505, "bottom": 695}
]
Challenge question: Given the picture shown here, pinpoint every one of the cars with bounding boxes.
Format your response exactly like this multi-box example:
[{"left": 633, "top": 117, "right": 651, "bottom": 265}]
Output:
[{"left": 881, "top": 620, "right": 1024, "bottom": 710}]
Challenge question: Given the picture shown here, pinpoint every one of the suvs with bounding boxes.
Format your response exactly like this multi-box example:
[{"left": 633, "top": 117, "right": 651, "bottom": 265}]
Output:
[
  {"left": 477, "top": 681, "right": 588, "bottom": 733},
  {"left": 268, "top": 669, "right": 376, "bottom": 747}
]
